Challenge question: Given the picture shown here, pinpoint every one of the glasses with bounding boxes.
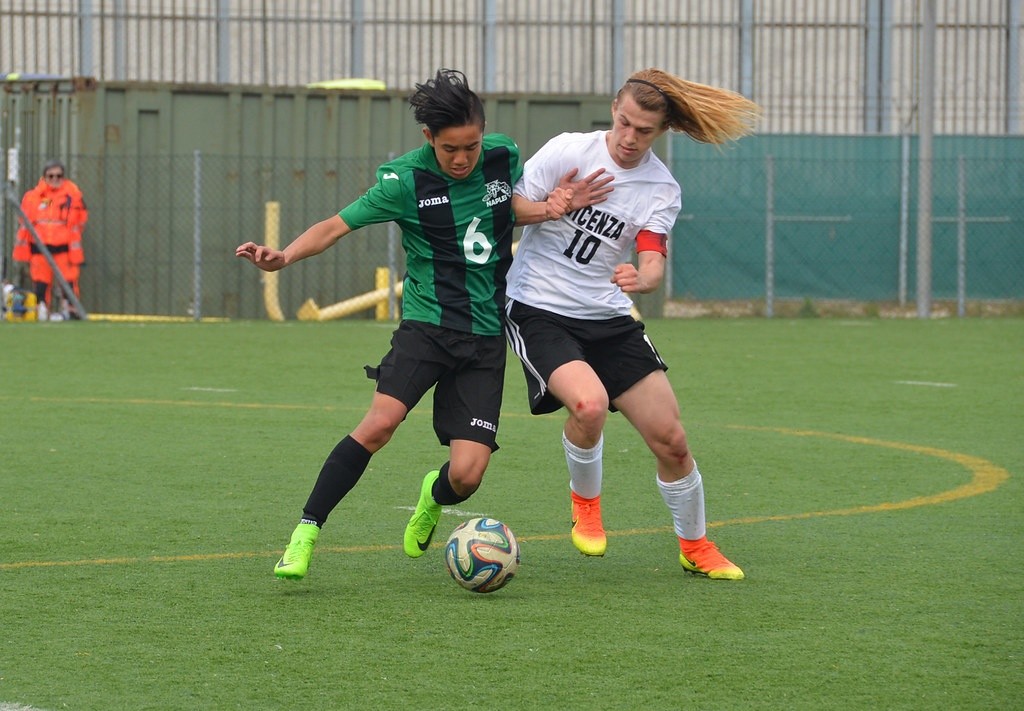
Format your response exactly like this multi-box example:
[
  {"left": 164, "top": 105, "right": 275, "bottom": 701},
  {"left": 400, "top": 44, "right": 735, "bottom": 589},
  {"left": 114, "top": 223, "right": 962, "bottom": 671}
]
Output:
[{"left": 45, "top": 175, "right": 63, "bottom": 180}]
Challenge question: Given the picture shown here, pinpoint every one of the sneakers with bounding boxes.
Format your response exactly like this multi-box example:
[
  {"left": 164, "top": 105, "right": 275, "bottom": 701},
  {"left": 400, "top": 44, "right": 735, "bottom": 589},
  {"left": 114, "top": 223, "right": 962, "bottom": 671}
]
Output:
[
  {"left": 273, "top": 522, "right": 320, "bottom": 581},
  {"left": 570, "top": 491, "right": 606, "bottom": 558},
  {"left": 404, "top": 470, "right": 442, "bottom": 558},
  {"left": 678, "top": 536, "right": 745, "bottom": 580}
]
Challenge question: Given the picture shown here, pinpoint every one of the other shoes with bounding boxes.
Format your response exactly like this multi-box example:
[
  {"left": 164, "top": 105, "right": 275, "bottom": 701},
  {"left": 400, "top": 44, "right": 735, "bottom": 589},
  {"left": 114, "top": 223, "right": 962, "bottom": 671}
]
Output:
[
  {"left": 62, "top": 300, "right": 70, "bottom": 320},
  {"left": 39, "top": 302, "right": 49, "bottom": 322}
]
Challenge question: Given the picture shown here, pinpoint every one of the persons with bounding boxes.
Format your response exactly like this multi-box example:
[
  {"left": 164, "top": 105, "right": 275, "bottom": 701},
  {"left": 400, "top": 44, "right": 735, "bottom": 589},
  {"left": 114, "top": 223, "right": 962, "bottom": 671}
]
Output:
[
  {"left": 505, "top": 66, "right": 768, "bottom": 580},
  {"left": 235, "top": 69, "right": 523, "bottom": 582},
  {"left": 13, "top": 163, "right": 88, "bottom": 320}
]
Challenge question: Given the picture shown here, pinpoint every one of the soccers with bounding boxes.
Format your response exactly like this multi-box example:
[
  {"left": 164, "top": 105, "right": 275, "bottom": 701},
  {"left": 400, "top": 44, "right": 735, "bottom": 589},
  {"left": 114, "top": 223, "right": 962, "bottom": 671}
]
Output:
[{"left": 445, "top": 517, "right": 521, "bottom": 593}]
